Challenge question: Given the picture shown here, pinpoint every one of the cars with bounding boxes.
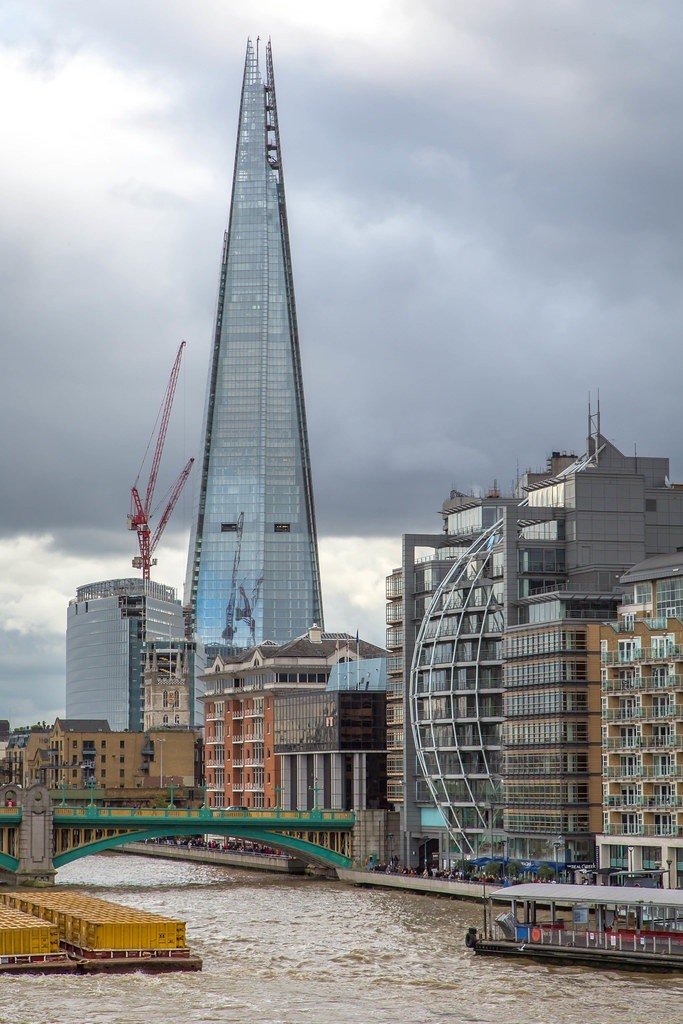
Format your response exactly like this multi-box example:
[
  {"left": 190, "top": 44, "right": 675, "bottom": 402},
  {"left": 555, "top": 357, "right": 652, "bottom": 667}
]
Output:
[{"left": 223, "top": 806, "right": 249, "bottom": 813}]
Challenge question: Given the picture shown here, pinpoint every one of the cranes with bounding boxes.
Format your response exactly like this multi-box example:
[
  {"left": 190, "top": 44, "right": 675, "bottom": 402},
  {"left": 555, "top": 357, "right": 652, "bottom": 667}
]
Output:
[
  {"left": 221, "top": 512, "right": 264, "bottom": 646},
  {"left": 127, "top": 340, "right": 194, "bottom": 579}
]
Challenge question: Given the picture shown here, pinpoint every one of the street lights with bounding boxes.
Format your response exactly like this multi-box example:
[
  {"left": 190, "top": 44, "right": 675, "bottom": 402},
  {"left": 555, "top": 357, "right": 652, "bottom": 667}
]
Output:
[
  {"left": 627, "top": 844, "right": 635, "bottom": 873},
  {"left": 554, "top": 842, "right": 561, "bottom": 884},
  {"left": 271, "top": 784, "right": 285, "bottom": 810},
  {"left": 459, "top": 837, "right": 466, "bottom": 881},
  {"left": 500, "top": 840, "right": 507, "bottom": 884},
  {"left": 56, "top": 777, "right": 74, "bottom": 806},
  {"left": 387, "top": 833, "right": 394, "bottom": 872},
  {"left": 85, "top": 778, "right": 97, "bottom": 808},
  {"left": 307, "top": 777, "right": 324, "bottom": 810},
  {"left": 198, "top": 784, "right": 215, "bottom": 809},
  {"left": 666, "top": 859, "right": 673, "bottom": 889},
  {"left": 423, "top": 836, "right": 429, "bottom": 877},
  {"left": 164, "top": 778, "right": 180, "bottom": 810},
  {"left": 156, "top": 737, "right": 167, "bottom": 788}
]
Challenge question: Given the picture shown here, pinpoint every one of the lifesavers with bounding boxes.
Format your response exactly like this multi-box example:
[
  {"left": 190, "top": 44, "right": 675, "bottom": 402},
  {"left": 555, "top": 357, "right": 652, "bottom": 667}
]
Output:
[{"left": 532, "top": 928, "right": 540, "bottom": 942}]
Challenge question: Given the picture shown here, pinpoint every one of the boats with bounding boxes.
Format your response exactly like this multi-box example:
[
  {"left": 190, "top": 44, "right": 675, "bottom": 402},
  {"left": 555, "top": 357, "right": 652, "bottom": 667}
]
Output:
[{"left": 464, "top": 875, "right": 682, "bottom": 968}]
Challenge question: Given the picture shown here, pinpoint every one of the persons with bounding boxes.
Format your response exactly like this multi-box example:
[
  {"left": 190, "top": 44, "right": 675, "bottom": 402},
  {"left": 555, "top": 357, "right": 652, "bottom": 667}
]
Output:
[
  {"left": 161, "top": 838, "right": 281, "bottom": 855},
  {"left": 367, "top": 863, "right": 557, "bottom": 888},
  {"left": 584, "top": 879, "right": 605, "bottom": 885}
]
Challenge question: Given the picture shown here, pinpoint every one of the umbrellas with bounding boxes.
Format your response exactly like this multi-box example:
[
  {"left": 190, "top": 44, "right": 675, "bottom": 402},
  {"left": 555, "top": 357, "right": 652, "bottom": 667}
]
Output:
[{"left": 467, "top": 857, "right": 543, "bottom": 871}]
